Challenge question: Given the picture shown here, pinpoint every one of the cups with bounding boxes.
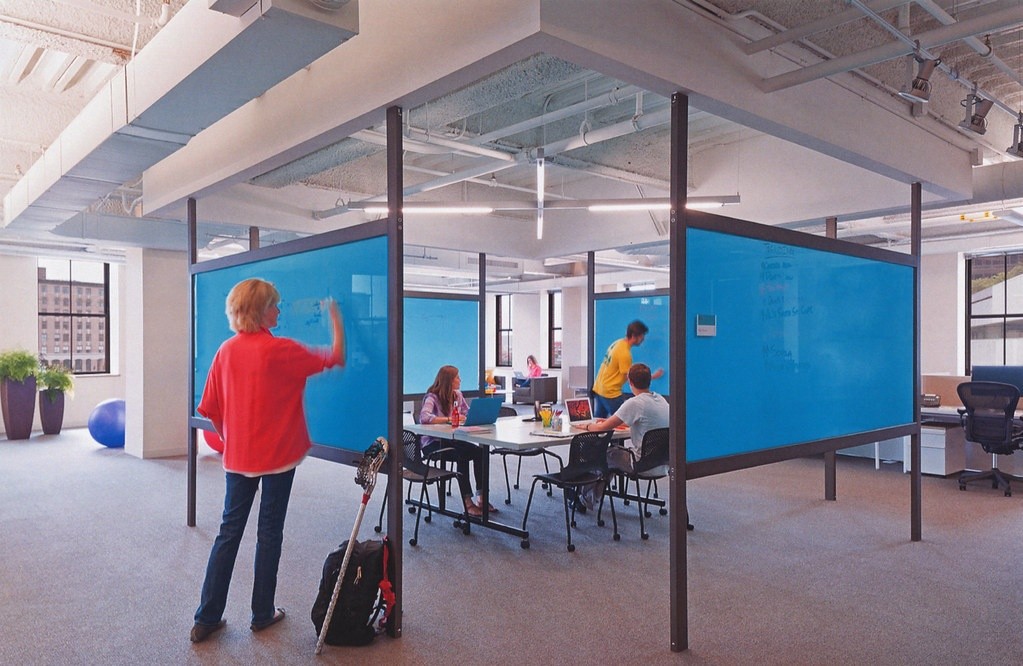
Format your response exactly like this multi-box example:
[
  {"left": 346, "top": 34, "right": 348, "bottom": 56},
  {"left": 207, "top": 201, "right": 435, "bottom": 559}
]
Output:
[
  {"left": 538, "top": 411, "right": 551, "bottom": 427},
  {"left": 550, "top": 416, "right": 562, "bottom": 431}
]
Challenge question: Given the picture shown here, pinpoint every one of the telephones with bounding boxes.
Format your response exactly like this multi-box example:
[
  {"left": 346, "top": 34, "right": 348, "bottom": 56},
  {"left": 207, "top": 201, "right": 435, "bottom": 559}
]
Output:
[
  {"left": 920, "top": 393, "right": 941, "bottom": 407},
  {"left": 534, "top": 400, "right": 552, "bottom": 421}
]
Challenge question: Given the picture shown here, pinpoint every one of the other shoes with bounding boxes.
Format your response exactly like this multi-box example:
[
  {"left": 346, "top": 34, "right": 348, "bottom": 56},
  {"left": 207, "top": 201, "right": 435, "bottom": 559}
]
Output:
[
  {"left": 249, "top": 606, "right": 285, "bottom": 632},
  {"left": 569, "top": 499, "right": 587, "bottom": 514},
  {"left": 191, "top": 618, "right": 227, "bottom": 643}
]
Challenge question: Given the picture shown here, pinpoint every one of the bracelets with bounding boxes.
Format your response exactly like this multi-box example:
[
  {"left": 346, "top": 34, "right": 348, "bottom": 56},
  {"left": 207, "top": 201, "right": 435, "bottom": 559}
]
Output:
[
  {"left": 587, "top": 424, "right": 589, "bottom": 430},
  {"left": 448, "top": 417, "right": 450, "bottom": 422}
]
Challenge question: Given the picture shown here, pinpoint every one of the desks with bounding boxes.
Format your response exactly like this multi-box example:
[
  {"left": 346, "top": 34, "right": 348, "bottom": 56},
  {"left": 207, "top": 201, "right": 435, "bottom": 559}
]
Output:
[
  {"left": 456, "top": 418, "right": 631, "bottom": 551},
  {"left": 904, "top": 404, "right": 1023, "bottom": 476},
  {"left": 486, "top": 390, "right": 517, "bottom": 398},
  {"left": 402, "top": 415, "right": 571, "bottom": 528},
  {"left": 567, "top": 366, "right": 588, "bottom": 400}
]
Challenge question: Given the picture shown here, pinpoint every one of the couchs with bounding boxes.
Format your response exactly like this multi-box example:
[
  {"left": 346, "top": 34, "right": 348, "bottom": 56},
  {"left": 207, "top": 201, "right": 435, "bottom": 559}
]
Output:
[{"left": 485, "top": 373, "right": 557, "bottom": 406}]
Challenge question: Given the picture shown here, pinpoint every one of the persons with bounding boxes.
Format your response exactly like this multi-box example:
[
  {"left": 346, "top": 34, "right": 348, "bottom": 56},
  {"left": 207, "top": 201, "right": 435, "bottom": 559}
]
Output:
[
  {"left": 516, "top": 355, "right": 542, "bottom": 388},
  {"left": 592, "top": 320, "right": 662, "bottom": 447},
  {"left": 190, "top": 279, "right": 344, "bottom": 645},
  {"left": 420, "top": 364, "right": 499, "bottom": 517},
  {"left": 569, "top": 363, "right": 670, "bottom": 514}
]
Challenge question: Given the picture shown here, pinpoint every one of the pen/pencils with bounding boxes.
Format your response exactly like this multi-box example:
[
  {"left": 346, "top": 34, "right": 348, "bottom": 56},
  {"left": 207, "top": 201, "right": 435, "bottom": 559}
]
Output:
[{"left": 539, "top": 409, "right": 563, "bottom": 426}]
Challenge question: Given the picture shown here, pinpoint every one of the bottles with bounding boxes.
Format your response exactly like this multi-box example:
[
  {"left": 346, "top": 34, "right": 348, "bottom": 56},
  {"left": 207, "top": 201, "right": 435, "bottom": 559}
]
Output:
[
  {"left": 541, "top": 404, "right": 552, "bottom": 410},
  {"left": 451, "top": 401, "right": 459, "bottom": 427},
  {"left": 534, "top": 401, "right": 541, "bottom": 420}
]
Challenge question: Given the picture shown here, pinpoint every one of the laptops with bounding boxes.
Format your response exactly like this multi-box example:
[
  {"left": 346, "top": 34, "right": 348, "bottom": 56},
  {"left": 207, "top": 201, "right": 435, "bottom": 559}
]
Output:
[
  {"left": 447, "top": 397, "right": 503, "bottom": 426},
  {"left": 565, "top": 397, "right": 597, "bottom": 426}
]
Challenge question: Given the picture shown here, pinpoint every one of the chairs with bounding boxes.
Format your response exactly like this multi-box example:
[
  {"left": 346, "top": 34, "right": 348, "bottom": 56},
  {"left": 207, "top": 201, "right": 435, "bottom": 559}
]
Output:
[
  {"left": 955, "top": 381, "right": 1023, "bottom": 496},
  {"left": 373, "top": 408, "right": 695, "bottom": 554}
]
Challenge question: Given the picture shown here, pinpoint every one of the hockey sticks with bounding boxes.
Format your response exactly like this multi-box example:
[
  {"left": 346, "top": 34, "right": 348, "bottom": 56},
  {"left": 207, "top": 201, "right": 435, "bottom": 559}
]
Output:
[{"left": 314, "top": 438, "right": 389, "bottom": 655}]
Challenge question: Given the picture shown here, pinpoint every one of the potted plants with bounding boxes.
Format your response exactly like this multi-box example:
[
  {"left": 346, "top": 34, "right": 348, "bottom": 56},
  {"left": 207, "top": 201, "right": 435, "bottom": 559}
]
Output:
[{"left": 0, "top": 352, "right": 73, "bottom": 439}]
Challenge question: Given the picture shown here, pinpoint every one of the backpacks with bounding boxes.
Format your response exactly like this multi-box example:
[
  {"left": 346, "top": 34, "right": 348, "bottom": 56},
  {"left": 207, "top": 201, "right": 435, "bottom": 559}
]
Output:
[{"left": 311, "top": 535, "right": 396, "bottom": 645}]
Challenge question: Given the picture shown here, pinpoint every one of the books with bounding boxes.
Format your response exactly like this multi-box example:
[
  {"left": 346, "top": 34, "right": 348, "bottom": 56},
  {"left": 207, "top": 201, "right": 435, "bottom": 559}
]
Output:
[{"left": 454, "top": 426, "right": 493, "bottom": 434}]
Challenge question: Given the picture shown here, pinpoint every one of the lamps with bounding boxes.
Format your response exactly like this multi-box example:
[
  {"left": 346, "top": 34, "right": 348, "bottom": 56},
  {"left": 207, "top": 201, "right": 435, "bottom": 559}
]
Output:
[
  {"left": 897, "top": 56, "right": 937, "bottom": 103},
  {"left": 1006, "top": 119, "right": 1023, "bottom": 159},
  {"left": 958, "top": 94, "right": 995, "bottom": 136},
  {"left": 347, "top": 129, "right": 740, "bottom": 241}
]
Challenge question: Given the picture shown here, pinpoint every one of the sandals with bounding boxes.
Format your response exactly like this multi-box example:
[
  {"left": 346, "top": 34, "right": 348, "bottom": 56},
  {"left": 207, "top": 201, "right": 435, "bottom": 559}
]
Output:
[{"left": 464, "top": 500, "right": 499, "bottom": 517}]
